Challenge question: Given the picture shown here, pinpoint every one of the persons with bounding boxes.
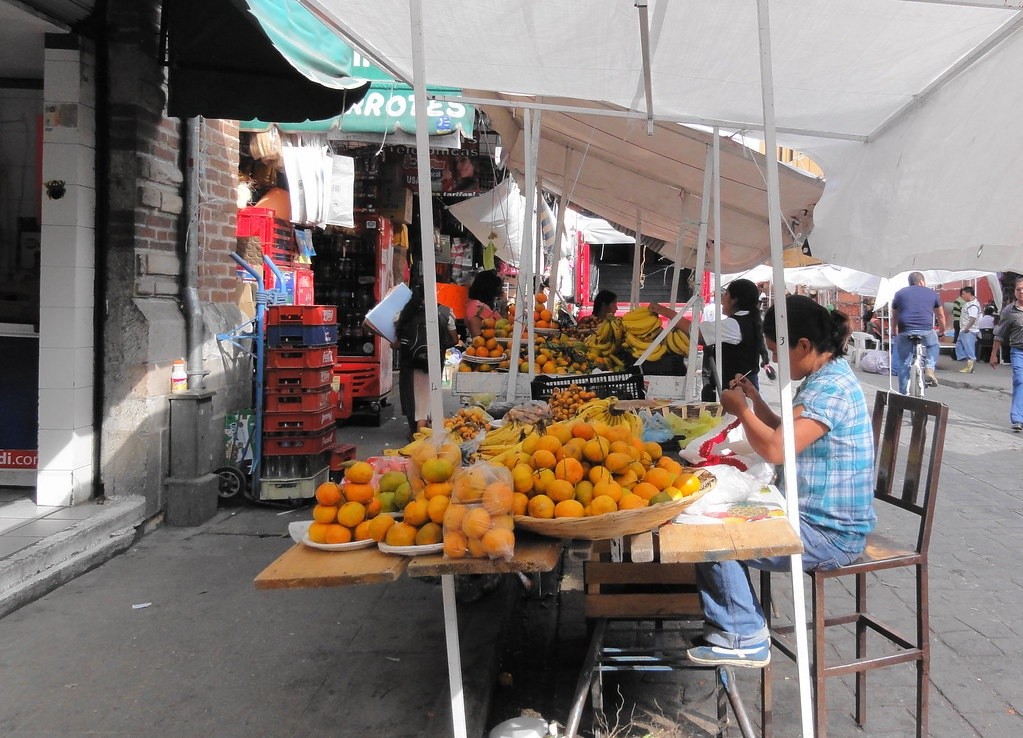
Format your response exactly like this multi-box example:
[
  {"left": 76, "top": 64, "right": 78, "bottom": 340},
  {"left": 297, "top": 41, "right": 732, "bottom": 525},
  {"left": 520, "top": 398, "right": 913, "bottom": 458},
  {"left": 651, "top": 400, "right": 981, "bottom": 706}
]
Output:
[
  {"left": 863, "top": 304, "right": 874, "bottom": 333},
  {"left": 889, "top": 272, "right": 947, "bottom": 394},
  {"left": 955, "top": 287, "right": 982, "bottom": 375},
  {"left": 647, "top": 279, "right": 775, "bottom": 401},
  {"left": 952, "top": 289, "right": 960, "bottom": 345},
  {"left": 391, "top": 300, "right": 458, "bottom": 442},
  {"left": 687, "top": 295, "right": 875, "bottom": 668},
  {"left": 471, "top": 269, "right": 504, "bottom": 309},
  {"left": 982, "top": 307, "right": 995, "bottom": 330},
  {"left": 590, "top": 290, "right": 619, "bottom": 318},
  {"left": 990, "top": 277, "right": 1022, "bottom": 430}
]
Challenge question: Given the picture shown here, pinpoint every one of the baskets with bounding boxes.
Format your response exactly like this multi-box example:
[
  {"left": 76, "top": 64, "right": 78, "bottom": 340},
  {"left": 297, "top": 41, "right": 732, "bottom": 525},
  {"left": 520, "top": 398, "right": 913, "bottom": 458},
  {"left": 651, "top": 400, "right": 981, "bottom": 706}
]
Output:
[
  {"left": 514, "top": 464, "right": 714, "bottom": 540},
  {"left": 263, "top": 305, "right": 337, "bottom": 455},
  {"left": 531, "top": 364, "right": 645, "bottom": 404}
]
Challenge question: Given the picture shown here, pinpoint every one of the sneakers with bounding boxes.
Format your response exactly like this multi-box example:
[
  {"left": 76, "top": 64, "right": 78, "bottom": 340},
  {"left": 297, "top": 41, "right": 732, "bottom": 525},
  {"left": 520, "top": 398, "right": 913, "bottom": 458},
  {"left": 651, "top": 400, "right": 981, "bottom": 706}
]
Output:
[{"left": 687, "top": 638, "right": 771, "bottom": 669}]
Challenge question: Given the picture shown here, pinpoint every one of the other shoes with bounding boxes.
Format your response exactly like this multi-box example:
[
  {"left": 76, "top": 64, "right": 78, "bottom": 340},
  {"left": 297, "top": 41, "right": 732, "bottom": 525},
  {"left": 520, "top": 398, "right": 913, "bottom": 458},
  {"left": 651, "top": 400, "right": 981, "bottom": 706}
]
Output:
[
  {"left": 960, "top": 367, "right": 969, "bottom": 373},
  {"left": 967, "top": 359, "right": 975, "bottom": 374},
  {"left": 924, "top": 370, "right": 938, "bottom": 387},
  {"left": 1012, "top": 422, "right": 1022, "bottom": 430}
]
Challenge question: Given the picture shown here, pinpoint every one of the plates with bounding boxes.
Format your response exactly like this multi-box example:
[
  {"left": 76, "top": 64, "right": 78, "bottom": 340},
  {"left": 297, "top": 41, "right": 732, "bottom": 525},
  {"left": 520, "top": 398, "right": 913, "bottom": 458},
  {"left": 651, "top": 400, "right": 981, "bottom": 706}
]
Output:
[
  {"left": 461, "top": 351, "right": 507, "bottom": 363},
  {"left": 301, "top": 531, "right": 375, "bottom": 551},
  {"left": 496, "top": 338, "right": 536, "bottom": 344},
  {"left": 489, "top": 420, "right": 503, "bottom": 427},
  {"left": 534, "top": 328, "right": 560, "bottom": 334},
  {"left": 378, "top": 540, "right": 444, "bottom": 556}
]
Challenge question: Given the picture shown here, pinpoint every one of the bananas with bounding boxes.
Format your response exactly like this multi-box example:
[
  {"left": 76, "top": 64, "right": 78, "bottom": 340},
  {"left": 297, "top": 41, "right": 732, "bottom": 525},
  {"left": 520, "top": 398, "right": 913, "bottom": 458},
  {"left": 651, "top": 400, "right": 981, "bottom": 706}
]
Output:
[
  {"left": 587, "top": 306, "right": 689, "bottom": 366},
  {"left": 383, "top": 396, "right": 644, "bottom": 466}
]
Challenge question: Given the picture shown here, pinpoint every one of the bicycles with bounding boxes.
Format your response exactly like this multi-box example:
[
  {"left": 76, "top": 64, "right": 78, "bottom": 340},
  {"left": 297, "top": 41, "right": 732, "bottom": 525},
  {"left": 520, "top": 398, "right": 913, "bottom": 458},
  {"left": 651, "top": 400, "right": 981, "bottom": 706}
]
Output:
[{"left": 891, "top": 333, "right": 945, "bottom": 428}]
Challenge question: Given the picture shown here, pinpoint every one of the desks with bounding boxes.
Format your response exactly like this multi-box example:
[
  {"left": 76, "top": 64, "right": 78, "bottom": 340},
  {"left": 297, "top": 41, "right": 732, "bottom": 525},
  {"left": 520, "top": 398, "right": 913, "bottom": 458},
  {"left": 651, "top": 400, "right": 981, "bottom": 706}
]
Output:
[{"left": 253, "top": 403, "right": 806, "bottom": 738}]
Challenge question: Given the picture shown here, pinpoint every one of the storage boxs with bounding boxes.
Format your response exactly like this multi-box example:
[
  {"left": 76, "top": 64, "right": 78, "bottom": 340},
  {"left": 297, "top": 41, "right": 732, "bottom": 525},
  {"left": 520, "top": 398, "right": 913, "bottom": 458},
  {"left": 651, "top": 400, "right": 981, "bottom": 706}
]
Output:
[
  {"left": 258, "top": 305, "right": 356, "bottom": 499},
  {"left": 530, "top": 364, "right": 645, "bottom": 402},
  {"left": 354, "top": 186, "right": 413, "bottom": 225}
]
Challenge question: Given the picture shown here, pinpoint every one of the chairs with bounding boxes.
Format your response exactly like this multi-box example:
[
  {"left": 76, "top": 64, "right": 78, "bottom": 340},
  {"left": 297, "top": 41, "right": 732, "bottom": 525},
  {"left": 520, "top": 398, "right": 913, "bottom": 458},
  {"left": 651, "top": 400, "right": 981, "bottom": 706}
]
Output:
[
  {"left": 759, "top": 389, "right": 950, "bottom": 738},
  {"left": 851, "top": 332, "right": 880, "bottom": 368},
  {"left": 975, "top": 328, "right": 1001, "bottom": 365}
]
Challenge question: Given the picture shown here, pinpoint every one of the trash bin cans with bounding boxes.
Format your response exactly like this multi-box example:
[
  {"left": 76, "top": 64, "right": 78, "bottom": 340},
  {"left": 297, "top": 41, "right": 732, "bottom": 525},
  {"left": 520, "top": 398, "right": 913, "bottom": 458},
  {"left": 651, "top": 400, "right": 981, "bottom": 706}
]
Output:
[{"left": 979, "top": 328, "right": 1000, "bottom": 363}]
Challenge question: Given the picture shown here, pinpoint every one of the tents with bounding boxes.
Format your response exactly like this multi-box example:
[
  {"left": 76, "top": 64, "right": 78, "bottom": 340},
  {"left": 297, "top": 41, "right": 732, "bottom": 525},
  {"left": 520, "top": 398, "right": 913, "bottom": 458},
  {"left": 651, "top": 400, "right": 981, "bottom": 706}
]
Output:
[{"left": 295, "top": 0, "right": 1023, "bottom": 737}]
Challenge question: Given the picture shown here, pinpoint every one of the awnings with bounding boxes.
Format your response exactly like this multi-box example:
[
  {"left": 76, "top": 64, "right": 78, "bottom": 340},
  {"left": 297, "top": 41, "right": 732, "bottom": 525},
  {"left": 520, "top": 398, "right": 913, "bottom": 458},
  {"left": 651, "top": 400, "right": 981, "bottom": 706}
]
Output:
[
  {"left": 119, "top": 0, "right": 371, "bottom": 110},
  {"left": 225, "top": 84, "right": 479, "bottom": 146}
]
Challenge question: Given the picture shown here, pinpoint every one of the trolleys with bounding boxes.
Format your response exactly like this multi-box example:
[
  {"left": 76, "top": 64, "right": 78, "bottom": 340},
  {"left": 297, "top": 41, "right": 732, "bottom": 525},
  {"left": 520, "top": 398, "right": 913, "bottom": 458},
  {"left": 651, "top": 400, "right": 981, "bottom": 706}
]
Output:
[{"left": 216, "top": 248, "right": 293, "bottom": 506}]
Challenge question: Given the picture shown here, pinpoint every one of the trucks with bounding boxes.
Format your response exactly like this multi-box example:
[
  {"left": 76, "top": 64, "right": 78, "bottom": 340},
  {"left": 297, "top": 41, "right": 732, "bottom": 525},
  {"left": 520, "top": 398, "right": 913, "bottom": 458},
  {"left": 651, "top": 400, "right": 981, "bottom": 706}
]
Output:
[{"left": 569, "top": 211, "right": 711, "bottom": 351}]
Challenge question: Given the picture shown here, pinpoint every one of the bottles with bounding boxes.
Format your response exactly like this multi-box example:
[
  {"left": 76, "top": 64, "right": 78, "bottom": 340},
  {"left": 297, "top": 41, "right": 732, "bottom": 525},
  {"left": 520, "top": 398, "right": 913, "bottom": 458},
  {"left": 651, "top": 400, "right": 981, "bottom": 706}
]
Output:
[
  {"left": 278, "top": 379, "right": 302, "bottom": 404},
  {"left": 334, "top": 303, "right": 374, "bottom": 348},
  {"left": 264, "top": 456, "right": 311, "bottom": 479},
  {"left": 171, "top": 360, "right": 188, "bottom": 395},
  {"left": 281, "top": 421, "right": 302, "bottom": 448},
  {"left": 280, "top": 336, "right": 303, "bottom": 358}
]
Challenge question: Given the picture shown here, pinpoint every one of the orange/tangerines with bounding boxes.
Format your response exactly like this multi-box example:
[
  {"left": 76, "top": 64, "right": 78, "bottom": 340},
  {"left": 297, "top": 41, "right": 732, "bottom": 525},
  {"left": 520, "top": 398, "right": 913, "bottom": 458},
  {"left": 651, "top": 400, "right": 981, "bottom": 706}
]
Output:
[
  {"left": 459, "top": 293, "right": 626, "bottom": 374},
  {"left": 308, "top": 421, "right": 701, "bottom": 560}
]
